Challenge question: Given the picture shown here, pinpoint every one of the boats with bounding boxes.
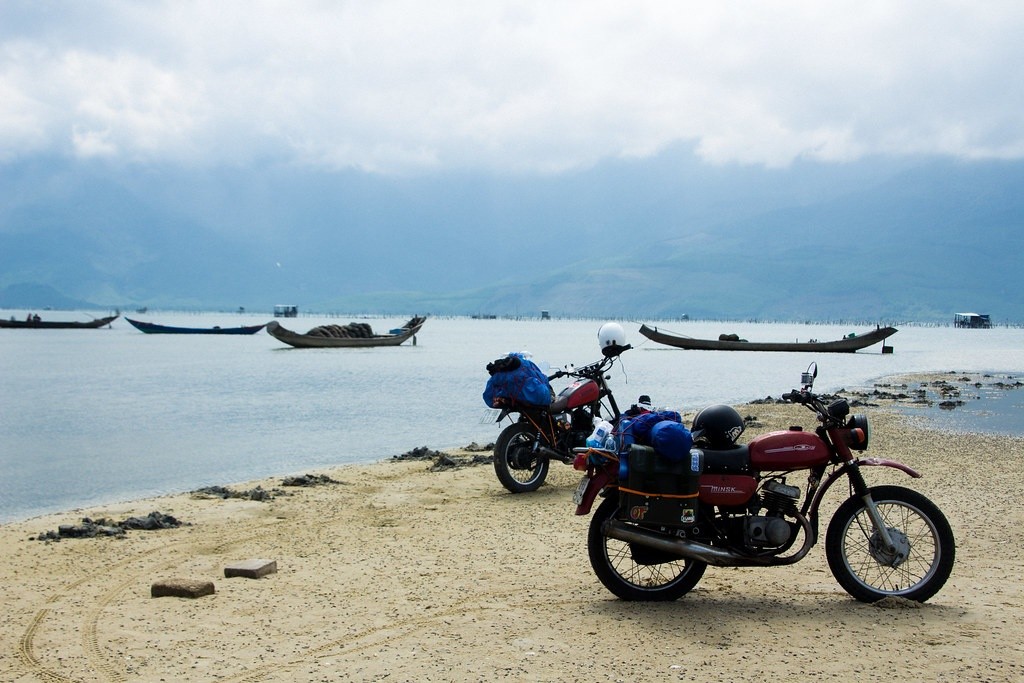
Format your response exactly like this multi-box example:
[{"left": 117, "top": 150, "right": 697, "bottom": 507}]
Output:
[
  {"left": 638, "top": 324, "right": 898, "bottom": 353},
  {"left": 125, "top": 315, "right": 268, "bottom": 336},
  {"left": 0, "top": 312, "right": 119, "bottom": 329},
  {"left": 266, "top": 314, "right": 428, "bottom": 348}
]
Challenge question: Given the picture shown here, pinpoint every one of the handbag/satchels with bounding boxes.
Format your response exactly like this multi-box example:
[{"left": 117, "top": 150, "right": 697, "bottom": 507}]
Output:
[
  {"left": 618, "top": 443, "right": 705, "bottom": 526},
  {"left": 483, "top": 353, "right": 552, "bottom": 406}
]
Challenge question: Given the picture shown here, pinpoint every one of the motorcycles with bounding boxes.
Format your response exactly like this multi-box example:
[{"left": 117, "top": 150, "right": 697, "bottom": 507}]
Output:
[
  {"left": 572, "top": 360, "right": 957, "bottom": 607},
  {"left": 482, "top": 322, "right": 633, "bottom": 494}
]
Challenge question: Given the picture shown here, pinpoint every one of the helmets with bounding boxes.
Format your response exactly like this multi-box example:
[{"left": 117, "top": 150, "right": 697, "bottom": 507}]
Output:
[{"left": 691, "top": 405, "right": 744, "bottom": 447}]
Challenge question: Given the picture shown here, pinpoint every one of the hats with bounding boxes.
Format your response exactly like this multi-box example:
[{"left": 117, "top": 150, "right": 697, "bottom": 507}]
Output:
[{"left": 597, "top": 323, "right": 625, "bottom": 356}]
